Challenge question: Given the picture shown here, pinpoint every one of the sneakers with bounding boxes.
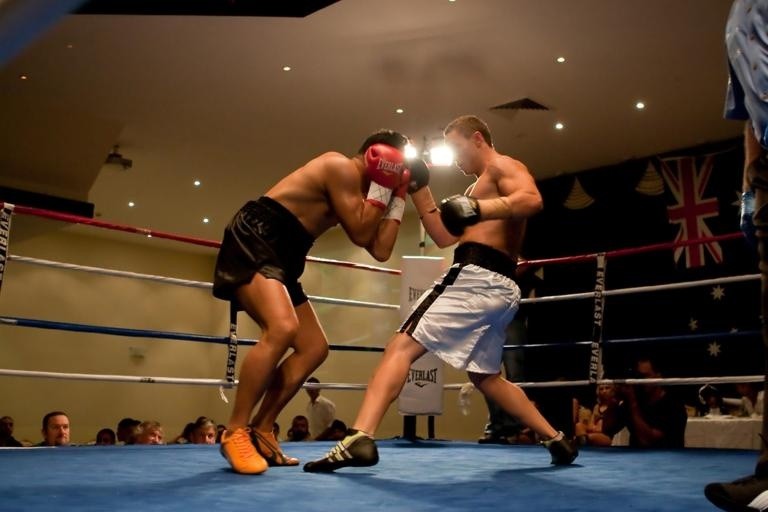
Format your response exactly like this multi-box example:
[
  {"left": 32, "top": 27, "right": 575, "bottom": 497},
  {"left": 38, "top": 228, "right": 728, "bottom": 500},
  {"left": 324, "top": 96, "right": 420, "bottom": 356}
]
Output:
[
  {"left": 221, "top": 427, "right": 299, "bottom": 474},
  {"left": 303, "top": 427, "right": 379, "bottom": 471},
  {"left": 704, "top": 454, "right": 768, "bottom": 511},
  {"left": 540, "top": 430, "right": 578, "bottom": 465}
]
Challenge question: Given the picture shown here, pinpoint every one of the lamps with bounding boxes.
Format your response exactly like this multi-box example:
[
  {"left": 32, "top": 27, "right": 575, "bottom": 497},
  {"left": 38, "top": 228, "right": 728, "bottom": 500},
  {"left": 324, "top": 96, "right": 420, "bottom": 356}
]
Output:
[{"left": 406, "top": 135, "right": 454, "bottom": 167}]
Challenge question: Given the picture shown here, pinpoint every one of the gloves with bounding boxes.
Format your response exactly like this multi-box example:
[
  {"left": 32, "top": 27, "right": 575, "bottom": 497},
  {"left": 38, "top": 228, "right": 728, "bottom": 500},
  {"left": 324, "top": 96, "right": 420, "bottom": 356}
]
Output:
[
  {"left": 363, "top": 143, "right": 430, "bottom": 223},
  {"left": 440, "top": 193, "right": 480, "bottom": 237}
]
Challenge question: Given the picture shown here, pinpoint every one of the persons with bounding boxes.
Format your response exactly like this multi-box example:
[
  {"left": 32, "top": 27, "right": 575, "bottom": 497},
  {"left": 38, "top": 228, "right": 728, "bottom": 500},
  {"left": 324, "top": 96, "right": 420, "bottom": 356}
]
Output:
[
  {"left": 91, "top": 375, "right": 349, "bottom": 447},
  {"left": 477, "top": 252, "right": 537, "bottom": 447},
  {"left": 502, "top": 343, "right": 768, "bottom": 450},
  {"left": 27, "top": 410, "right": 74, "bottom": 448},
  {"left": 210, "top": 126, "right": 419, "bottom": 476},
  {"left": 699, "top": 1, "right": 767, "bottom": 512},
  {"left": 297, "top": 113, "right": 582, "bottom": 473},
  {"left": 0, "top": 417, "right": 24, "bottom": 447}
]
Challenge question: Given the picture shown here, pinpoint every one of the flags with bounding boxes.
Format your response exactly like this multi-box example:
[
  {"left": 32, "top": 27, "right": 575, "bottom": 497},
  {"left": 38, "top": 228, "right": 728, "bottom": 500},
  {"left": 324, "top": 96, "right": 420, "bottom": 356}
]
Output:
[{"left": 661, "top": 149, "right": 723, "bottom": 268}]
